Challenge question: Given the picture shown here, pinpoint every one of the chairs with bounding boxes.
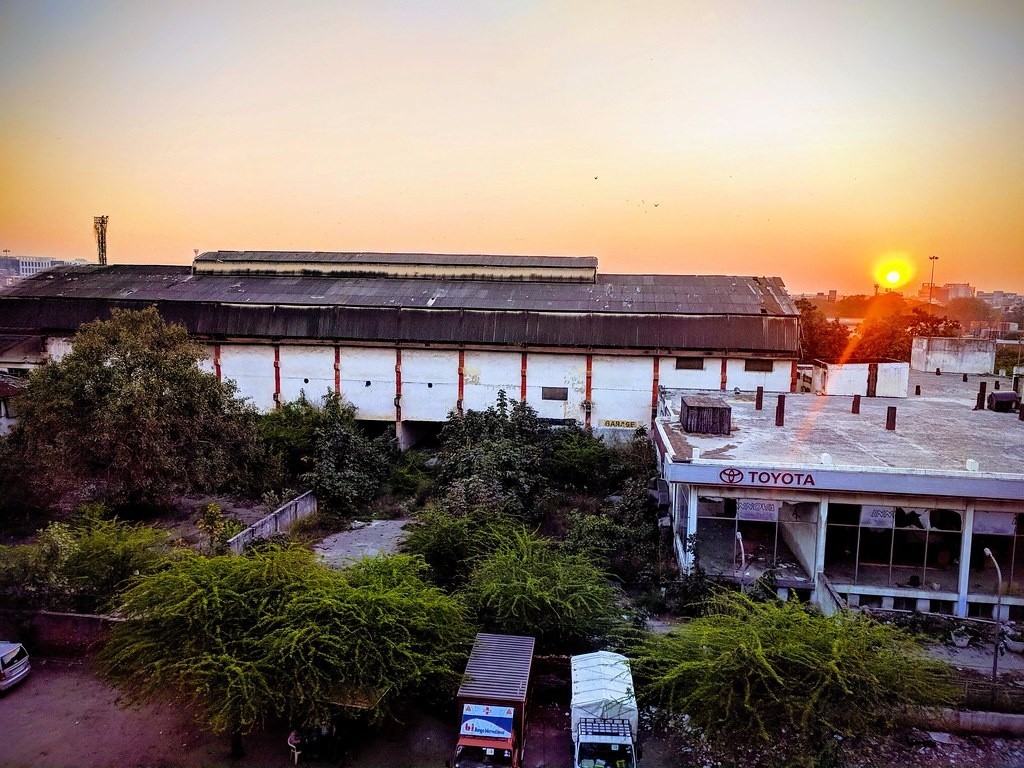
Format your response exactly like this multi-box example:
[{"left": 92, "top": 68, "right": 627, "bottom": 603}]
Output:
[{"left": 288, "top": 737, "right": 302, "bottom": 765}]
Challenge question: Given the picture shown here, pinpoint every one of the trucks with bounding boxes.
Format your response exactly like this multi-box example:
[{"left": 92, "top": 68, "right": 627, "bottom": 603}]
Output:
[
  {"left": 568, "top": 649, "right": 641, "bottom": 768},
  {"left": 448, "top": 632, "right": 534, "bottom": 768}
]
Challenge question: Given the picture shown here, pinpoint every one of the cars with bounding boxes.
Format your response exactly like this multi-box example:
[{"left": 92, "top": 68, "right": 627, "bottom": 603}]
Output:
[{"left": 0, "top": 637, "right": 32, "bottom": 694}]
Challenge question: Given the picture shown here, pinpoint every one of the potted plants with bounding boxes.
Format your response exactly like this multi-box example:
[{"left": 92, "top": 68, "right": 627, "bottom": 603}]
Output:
[
  {"left": 950, "top": 631, "right": 971, "bottom": 646},
  {"left": 1004, "top": 634, "right": 1024, "bottom": 653}
]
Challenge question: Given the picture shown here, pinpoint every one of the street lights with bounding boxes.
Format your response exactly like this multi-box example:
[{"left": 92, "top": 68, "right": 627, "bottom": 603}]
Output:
[
  {"left": 983, "top": 548, "right": 1004, "bottom": 709},
  {"left": 735, "top": 531, "right": 746, "bottom": 595},
  {"left": 926, "top": 254, "right": 939, "bottom": 323}
]
